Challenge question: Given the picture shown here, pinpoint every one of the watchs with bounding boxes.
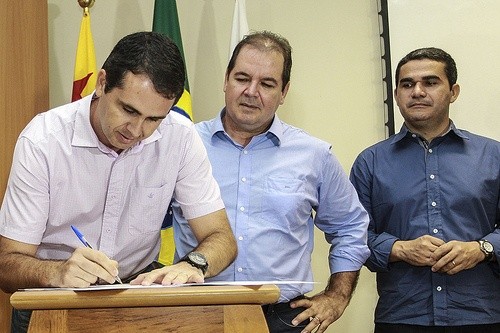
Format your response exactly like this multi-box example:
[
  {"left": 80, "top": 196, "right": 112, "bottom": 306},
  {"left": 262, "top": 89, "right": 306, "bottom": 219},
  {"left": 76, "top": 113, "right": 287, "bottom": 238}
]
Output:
[
  {"left": 179, "top": 252, "right": 209, "bottom": 275},
  {"left": 477, "top": 239, "right": 494, "bottom": 263}
]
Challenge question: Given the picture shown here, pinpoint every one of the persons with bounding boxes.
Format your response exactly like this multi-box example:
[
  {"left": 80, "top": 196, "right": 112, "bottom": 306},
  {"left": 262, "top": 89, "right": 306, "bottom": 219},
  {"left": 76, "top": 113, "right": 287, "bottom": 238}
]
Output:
[
  {"left": 0, "top": 32, "right": 239, "bottom": 333},
  {"left": 350, "top": 47, "right": 500, "bottom": 333},
  {"left": 172, "top": 31, "right": 370, "bottom": 333}
]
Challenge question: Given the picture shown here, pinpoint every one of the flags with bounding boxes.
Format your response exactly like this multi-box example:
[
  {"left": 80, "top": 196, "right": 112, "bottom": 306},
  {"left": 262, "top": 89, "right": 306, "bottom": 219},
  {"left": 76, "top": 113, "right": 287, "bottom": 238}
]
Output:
[
  {"left": 152, "top": 0, "right": 193, "bottom": 267},
  {"left": 230, "top": 0, "right": 249, "bottom": 63},
  {"left": 72, "top": 12, "right": 99, "bottom": 103}
]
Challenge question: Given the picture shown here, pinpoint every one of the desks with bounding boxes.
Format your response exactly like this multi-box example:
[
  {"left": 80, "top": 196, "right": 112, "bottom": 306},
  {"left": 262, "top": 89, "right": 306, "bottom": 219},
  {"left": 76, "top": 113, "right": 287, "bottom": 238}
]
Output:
[{"left": 9, "top": 284, "right": 281, "bottom": 333}]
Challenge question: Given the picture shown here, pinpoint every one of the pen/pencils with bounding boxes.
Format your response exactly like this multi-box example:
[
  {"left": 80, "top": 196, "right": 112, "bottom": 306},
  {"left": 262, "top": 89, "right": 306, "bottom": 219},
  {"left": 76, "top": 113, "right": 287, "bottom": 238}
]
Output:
[{"left": 71, "top": 225, "right": 123, "bottom": 285}]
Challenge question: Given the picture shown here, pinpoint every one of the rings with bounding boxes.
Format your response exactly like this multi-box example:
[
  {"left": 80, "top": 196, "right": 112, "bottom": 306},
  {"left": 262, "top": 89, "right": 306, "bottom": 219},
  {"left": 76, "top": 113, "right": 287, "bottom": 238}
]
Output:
[
  {"left": 451, "top": 260, "right": 456, "bottom": 267},
  {"left": 313, "top": 316, "right": 320, "bottom": 323}
]
